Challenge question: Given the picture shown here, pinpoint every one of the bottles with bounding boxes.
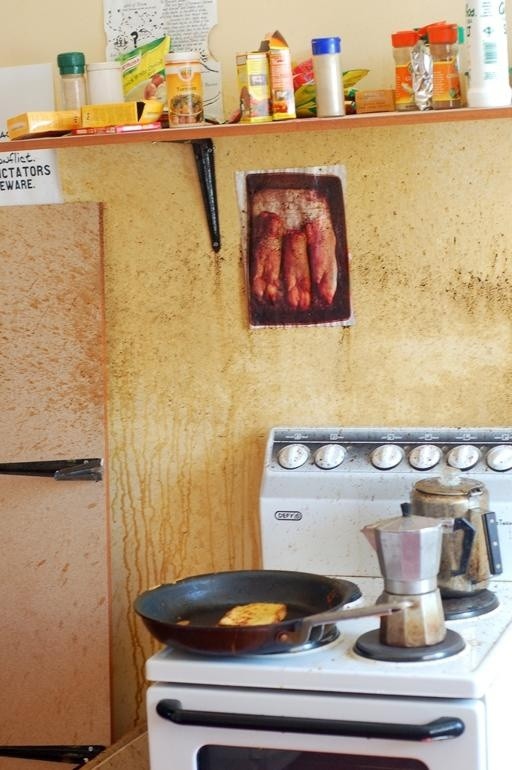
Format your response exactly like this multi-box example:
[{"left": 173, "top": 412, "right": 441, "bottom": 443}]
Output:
[
  {"left": 427, "top": 24, "right": 460, "bottom": 109},
  {"left": 163, "top": 53, "right": 205, "bottom": 129},
  {"left": 57, "top": 53, "right": 87, "bottom": 115},
  {"left": 392, "top": 32, "right": 425, "bottom": 112},
  {"left": 466, "top": 0, "right": 512, "bottom": 109},
  {"left": 312, "top": 37, "right": 345, "bottom": 119},
  {"left": 86, "top": 62, "right": 125, "bottom": 108}
]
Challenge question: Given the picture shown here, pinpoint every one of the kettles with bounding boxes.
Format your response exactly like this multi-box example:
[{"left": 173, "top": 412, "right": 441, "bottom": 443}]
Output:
[
  {"left": 361, "top": 503, "right": 476, "bottom": 663},
  {"left": 401, "top": 468, "right": 504, "bottom": 599}
]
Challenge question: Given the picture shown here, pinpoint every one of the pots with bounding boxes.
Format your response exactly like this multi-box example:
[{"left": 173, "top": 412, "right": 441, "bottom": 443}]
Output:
[{"left": 129, "top": 566, "right": 417, "bottom": 657}]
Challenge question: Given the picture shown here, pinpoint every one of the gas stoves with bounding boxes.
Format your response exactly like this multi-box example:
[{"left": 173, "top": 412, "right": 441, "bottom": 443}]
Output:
[{"left": 142, "top": 425, "right": 511, "bottom": 700}]
